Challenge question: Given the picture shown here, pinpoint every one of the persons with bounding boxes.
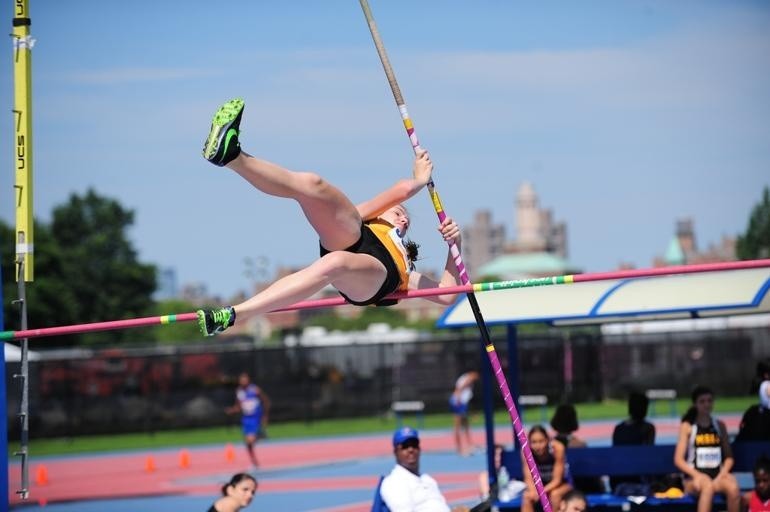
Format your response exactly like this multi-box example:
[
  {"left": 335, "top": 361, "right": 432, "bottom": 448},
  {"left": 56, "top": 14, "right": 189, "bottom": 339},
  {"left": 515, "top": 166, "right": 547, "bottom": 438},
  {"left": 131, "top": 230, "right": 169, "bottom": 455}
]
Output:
[
  {"left": 448, "top": 366, "right": 482, "bottom": 458},
  {"left": 226, "top": 372, "right": 272, "bottom": 475},
  {"left": 192, "top": 95, "right": 465, "bottom": 343},
  {"left": 481, "top": 360, "right": 770, "bottom": 512},
  {"left": 367, "top": 426, "right": 470, "bottom": 512},
  {"left": 205, "top": 473, "right": 258, "bottom": 512}
]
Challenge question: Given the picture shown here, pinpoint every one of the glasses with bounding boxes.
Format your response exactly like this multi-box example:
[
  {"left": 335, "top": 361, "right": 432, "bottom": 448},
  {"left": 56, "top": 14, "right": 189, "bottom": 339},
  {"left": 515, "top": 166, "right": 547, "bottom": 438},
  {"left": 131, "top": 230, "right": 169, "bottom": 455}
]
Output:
[{"left": 399, "top": 442, "right": 419, "bottom": 450}]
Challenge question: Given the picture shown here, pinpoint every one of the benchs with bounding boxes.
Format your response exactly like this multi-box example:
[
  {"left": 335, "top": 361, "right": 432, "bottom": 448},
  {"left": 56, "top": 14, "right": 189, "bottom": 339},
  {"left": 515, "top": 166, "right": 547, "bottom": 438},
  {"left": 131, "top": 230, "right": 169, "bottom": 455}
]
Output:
[{"left": 499, "top": 444, "right": 770, "bottom": 512}]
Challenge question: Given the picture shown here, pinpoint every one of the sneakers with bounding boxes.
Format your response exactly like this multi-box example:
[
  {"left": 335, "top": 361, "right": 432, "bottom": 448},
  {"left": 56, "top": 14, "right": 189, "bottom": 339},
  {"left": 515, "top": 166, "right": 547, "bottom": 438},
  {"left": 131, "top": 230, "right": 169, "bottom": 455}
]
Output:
[
  {"left": 200, "top": 96, "right": 247, "bottom": 168},
  {"left": 196, "top": 304, "right": 237, "bottom": 338}
]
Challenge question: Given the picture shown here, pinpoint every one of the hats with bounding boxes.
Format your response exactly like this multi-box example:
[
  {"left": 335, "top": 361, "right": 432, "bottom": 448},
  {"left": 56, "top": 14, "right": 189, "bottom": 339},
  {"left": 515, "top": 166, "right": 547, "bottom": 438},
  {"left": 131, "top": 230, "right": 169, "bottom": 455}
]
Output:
[{"left": 392, "top": 424, "right": 419, "bottom": 446}]
